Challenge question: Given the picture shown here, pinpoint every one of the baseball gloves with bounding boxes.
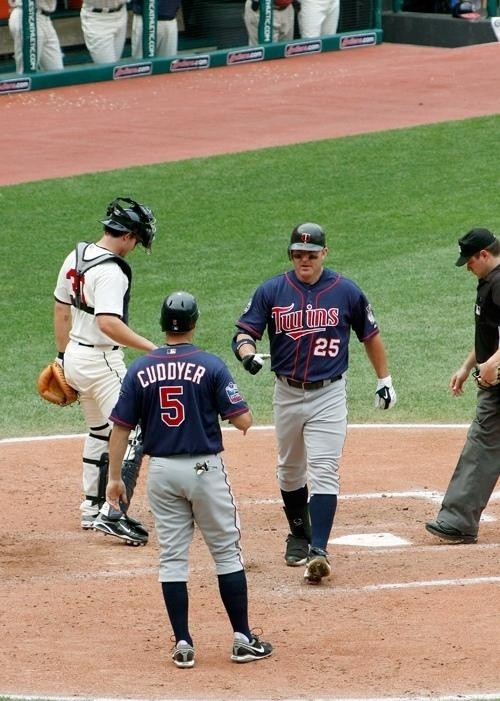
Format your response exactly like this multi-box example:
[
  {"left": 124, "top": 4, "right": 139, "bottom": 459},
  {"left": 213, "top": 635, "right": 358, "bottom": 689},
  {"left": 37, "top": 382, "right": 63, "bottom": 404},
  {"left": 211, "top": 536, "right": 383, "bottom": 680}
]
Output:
[{"left": 38, "top": 362, "right": 81, "bottom": 408}]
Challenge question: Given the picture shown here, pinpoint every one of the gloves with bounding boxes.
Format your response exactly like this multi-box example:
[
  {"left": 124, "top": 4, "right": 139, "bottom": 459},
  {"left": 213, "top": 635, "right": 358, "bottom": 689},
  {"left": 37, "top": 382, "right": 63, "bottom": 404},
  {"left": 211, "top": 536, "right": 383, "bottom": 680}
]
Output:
[
  {"left": 243, "top": 350, "right": 273, "bottom": 375},
  {"left": 374, "top": 374, "right": 399, "bottom": 411}
]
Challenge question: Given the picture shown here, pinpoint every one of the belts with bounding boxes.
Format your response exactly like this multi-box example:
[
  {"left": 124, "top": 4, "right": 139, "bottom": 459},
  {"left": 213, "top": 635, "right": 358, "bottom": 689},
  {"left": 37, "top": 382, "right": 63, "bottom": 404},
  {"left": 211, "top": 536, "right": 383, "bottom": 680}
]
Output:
[
  {"left": 86, "top": 5, "right": 126, "bottom": 13},
  {"left": 276, "top": 375, "right": 344, "bottom": 390}
]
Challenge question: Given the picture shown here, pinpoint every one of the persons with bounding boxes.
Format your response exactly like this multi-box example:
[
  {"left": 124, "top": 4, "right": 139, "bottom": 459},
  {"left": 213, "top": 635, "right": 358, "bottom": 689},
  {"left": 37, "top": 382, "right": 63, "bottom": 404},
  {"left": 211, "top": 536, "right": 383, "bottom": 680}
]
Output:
[
  {"left": 131, "top": 0, "right": 180, "bottom": 57},
  {"left": 245, "top": 0, "right": 295, "bottom": 46},
  {"left": 230, "top": 223, "right": 396, "bottom": 583},
  {"left": 426, "top": 228, "right": 500, "bottom": 544},
  {"left": 105, "top": 291, "right": 273, "bottom": 668},
  {"left": 37, "top": 196, "right": 158, "bottom": 541},
  {"left": 9, "top": 0, "right": 64, "bottom": 73},
  {"left": 297, "top": 0, "right": 340, "bottom": 38},
  {"left": 80, "top": 0, "right": 131, "bottom": 63}
]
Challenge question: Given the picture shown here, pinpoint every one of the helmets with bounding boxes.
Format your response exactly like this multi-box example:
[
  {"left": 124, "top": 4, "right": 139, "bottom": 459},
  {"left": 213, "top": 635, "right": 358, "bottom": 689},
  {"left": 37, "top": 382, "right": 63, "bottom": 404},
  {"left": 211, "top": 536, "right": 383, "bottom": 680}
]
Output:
[
  {"left": 286, "top": 219, "right": 328, "bottom": 254},
  {"left": 160, "top": 289, "right": 200, "bottom": 334},
  {"left": 99, "top": 195, "right": 156, "bottom": 251}
]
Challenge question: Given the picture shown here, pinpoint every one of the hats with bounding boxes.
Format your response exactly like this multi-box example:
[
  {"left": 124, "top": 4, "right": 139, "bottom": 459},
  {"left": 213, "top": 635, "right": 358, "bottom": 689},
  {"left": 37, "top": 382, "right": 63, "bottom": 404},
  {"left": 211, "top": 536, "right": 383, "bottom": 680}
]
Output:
[
  {"left": 455, "top": 227, "right": 498, "bottom": 266},
  {"left": 452, "top": 1, "right": 481, "bottom": 20}
]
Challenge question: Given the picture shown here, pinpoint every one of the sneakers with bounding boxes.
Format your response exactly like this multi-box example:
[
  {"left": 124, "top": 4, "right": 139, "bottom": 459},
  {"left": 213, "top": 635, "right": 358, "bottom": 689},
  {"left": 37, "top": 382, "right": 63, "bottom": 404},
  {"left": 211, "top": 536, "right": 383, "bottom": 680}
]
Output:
[
  {"left": 230, "top": 629, "right": 275, "bottom": 663},
  {"left": 79, "top": 495, "right": 152, "bottom": 546},
  {"left": 301, "top": 549, "right": 331, "bottom": 585},
  {"left": 168, "top": 640, "right": 198, "bottom": 670},
  {"left": 281, "top": 533, "right": 310, "bottom": 566},
  {"left": 424, "top": 517, "right": 479, "bottom": 546}
]
